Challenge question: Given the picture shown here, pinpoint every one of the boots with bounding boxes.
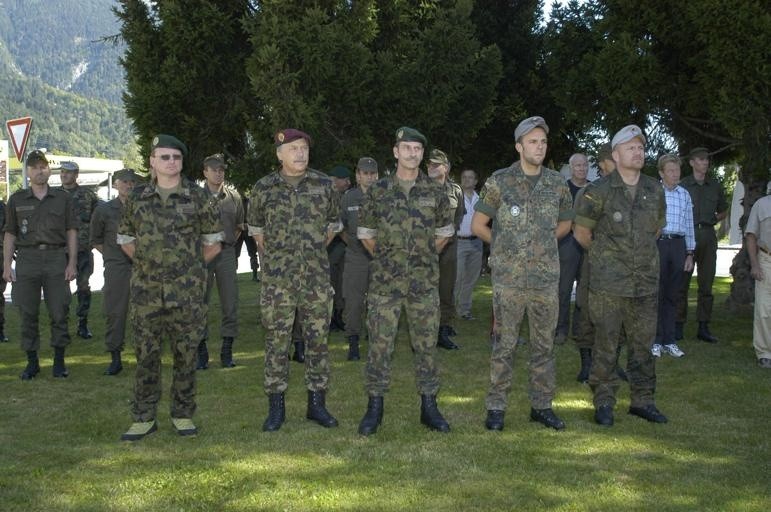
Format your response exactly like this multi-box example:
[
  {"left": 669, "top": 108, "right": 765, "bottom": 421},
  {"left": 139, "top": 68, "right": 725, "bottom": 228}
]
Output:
[
  {"left": 420, "top": 394, "right": 451, "bottom": 433},
  {"left": 348, "top": 335, "right": 359, "bottom": 360},
  {"left": 675, "top": 322, "right": 683, "bottom": 340},
  {"left": 291, "top": 341, "right": 305, "bottom": 362},
  {"left": 0, "top": 325, "right": 93, "bottom": 380},
  {"left": 103, "top": 351, "right": 122, "bottom": 375},
  {"left": 195, "top": 337, "right": 236, "bottom": 369},
  {"left": 438, "top": 313, "right": 478, "bottom": 349},
  {"left": 357, "top": 395, "right": 383, "bottom": 436},
  {"left": 577, "top": 346, "right": 627, "bottom": 384},
  {"left": 698, "top": 322, "right": 719, "bottom": 344},
  {"left": 262, "top": 392, "right": 339, "bottom": 431}
]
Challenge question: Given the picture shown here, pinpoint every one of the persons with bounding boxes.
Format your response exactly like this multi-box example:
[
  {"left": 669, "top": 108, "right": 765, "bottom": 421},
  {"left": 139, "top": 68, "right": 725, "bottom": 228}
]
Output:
[
  {"left": 194, "top": 154, "right": 260, "bottom": 371},
  {"left": 118, "top": 135, "right": 226, "bottom": 441},
  {"left": 570, "top": 123, "right": 671, "bottom": 427},
  {"left": 745, "top": 194, "right": 771, "bottom": 369},
  {"left": 288, "top": 149, "right": 485, "bottom": 363},
  {"left": 548, "top": 142, "right": 730, "bottom": 357},
  {"left": 469, "top": 114, "right": 572, "bottom": 432},
  {"left": 90, "top": 169, "right": 137, "bottom": 375},
  {"left": 0, "top": 147, "right": 99, "bottom": 379},
  {"left": 356, "top": 125, "right": 453, "bottom": 433},
  {"left": 247, "top": 129, "right": 345, "bottom": 430}
]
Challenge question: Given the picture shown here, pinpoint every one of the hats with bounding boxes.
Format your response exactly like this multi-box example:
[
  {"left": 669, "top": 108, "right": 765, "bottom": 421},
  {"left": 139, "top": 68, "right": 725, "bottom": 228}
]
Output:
[
  {"left": 26, "top": 134, "right": 227, "bottom": 181},
  {"left": 611, "top": 125, "right": 646, "bottom": 149},
  {"left": 274, "top": 128, "right": 451, "bottom": 178},
  {"left": 686, "top": 147, "right": 710, "bottom": 157},
  {"left": 514, "top": 116, "right": 550, "bottom": 141}
]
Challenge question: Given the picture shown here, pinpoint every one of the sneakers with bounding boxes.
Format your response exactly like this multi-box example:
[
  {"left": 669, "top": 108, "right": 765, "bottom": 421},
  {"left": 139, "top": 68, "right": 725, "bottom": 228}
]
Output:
[
  {"left": 758, "top": 358, "right": 771, "bottom": 368},
  {"left": 485, "top": 405, "right": 667, "bottom": 431},
  {"left": 121, "top": 420, "right": 157, "bottom": 441},
  {"left": 170, "top": 417, "right": 198, "bottom": 436},
  {"left": 650, "top": 343, "right": 685, "bottom": 358}
]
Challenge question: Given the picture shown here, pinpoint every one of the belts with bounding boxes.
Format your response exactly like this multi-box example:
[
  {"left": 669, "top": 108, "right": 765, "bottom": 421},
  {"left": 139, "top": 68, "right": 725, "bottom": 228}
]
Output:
[
  {"left": 759, "top": 247, "right": 771, "bottom": 256},
  {"left": 659, "top": 234, "right": 684, "bottom": 240},
  {"left": 24, "top": 244, "right": 64, "bottom": 249},
  {"left": 457, "top": 235, "right": 478, "bottom": 240}
]
